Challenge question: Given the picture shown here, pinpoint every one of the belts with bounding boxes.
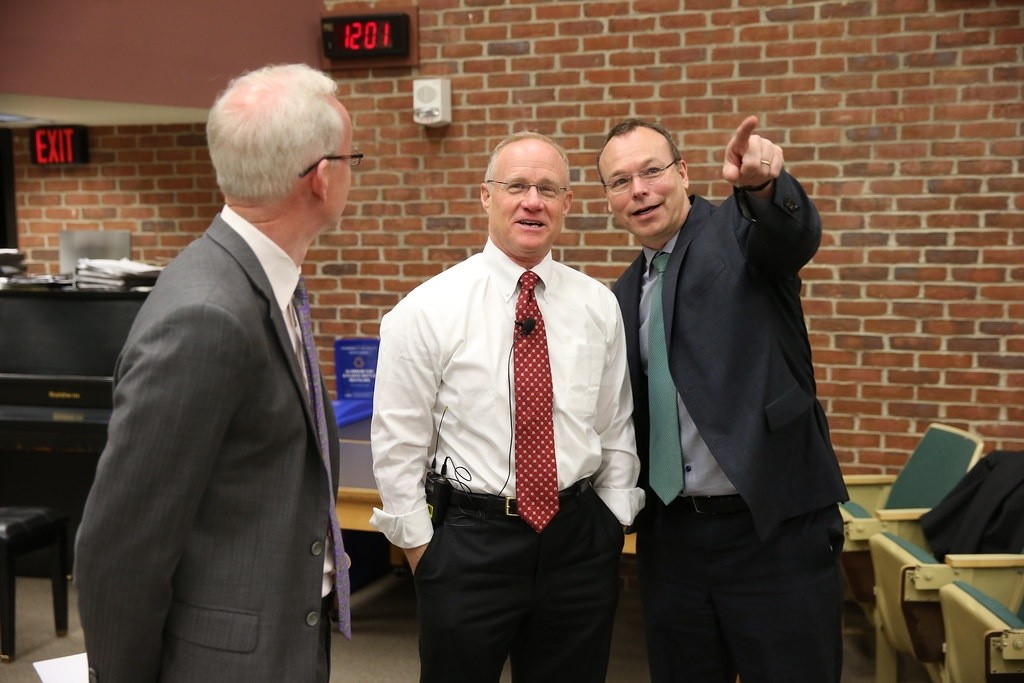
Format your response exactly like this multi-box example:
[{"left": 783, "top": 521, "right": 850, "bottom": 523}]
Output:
[
  {"left": 662, "top": 496, "right": 748, "bottom": 516},
  {"left": 451, "top": 484, "right": 585, "bottom": 518}
]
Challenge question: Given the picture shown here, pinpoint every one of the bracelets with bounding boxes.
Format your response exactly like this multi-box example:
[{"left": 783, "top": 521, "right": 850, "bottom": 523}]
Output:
[{"left": 745, "top": 179, "right": 771, "bottom": 192}]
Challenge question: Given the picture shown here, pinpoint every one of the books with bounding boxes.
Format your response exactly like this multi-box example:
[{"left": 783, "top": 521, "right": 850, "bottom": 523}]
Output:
[{"left": 74, "top": 261, "right": 161, "bottom": 292}]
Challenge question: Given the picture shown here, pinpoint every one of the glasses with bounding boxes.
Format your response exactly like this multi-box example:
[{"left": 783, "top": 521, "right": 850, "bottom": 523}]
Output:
[
  {"left": 602, "top": 159, "right": 678, "bottom": 190},
  {"left": 484, "top": 176, "right": 568, "bottom": 200},
  {"left": 297, "top": 148, "right": 362, "bottom": 177}
]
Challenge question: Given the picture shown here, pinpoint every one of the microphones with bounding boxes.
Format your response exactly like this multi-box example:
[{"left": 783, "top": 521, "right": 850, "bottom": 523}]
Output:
[{"left": 522, "top": 318, "right": 536, "bottom": 335}]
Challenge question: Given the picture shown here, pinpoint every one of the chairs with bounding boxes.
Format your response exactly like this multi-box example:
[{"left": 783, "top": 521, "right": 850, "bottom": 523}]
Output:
[
  {"left": 831, "top": 422, "right": 1024, "bottom": 683},
  {"left": 0, "top": 506, "right": 69, "bottom": 665}
]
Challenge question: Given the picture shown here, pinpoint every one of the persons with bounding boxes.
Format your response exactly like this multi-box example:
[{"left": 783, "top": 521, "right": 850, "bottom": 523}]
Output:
[
  {"left": 369, "top": 131, "right": 645, "bottom": 683},
  {"left": 597, "top": 115, "right": 851, "bottom": 682},
  {"left": 73, "top": 64, "right": 364, "bottom": 683}
]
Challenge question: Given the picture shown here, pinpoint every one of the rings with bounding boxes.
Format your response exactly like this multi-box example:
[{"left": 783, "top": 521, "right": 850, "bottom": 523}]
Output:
[{"left": 761, "top": 160, "right": 770, "bottom": 166}]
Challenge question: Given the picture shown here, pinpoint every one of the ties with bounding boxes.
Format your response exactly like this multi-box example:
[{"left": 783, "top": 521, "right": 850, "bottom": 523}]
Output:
[
  {"left": 648, "top": 250, "right": 687, "bottom": 505},
  {"left": 293, "top": 278, "right": 351, "bottom": 650},
  {"left": 513, "top": 272, "right": 561, "bottom": 528}
]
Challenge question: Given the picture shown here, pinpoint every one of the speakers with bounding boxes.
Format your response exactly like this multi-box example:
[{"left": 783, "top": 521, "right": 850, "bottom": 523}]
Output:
[{"left": 413, "top": 79, "right": 452, "bottom": 128}]
